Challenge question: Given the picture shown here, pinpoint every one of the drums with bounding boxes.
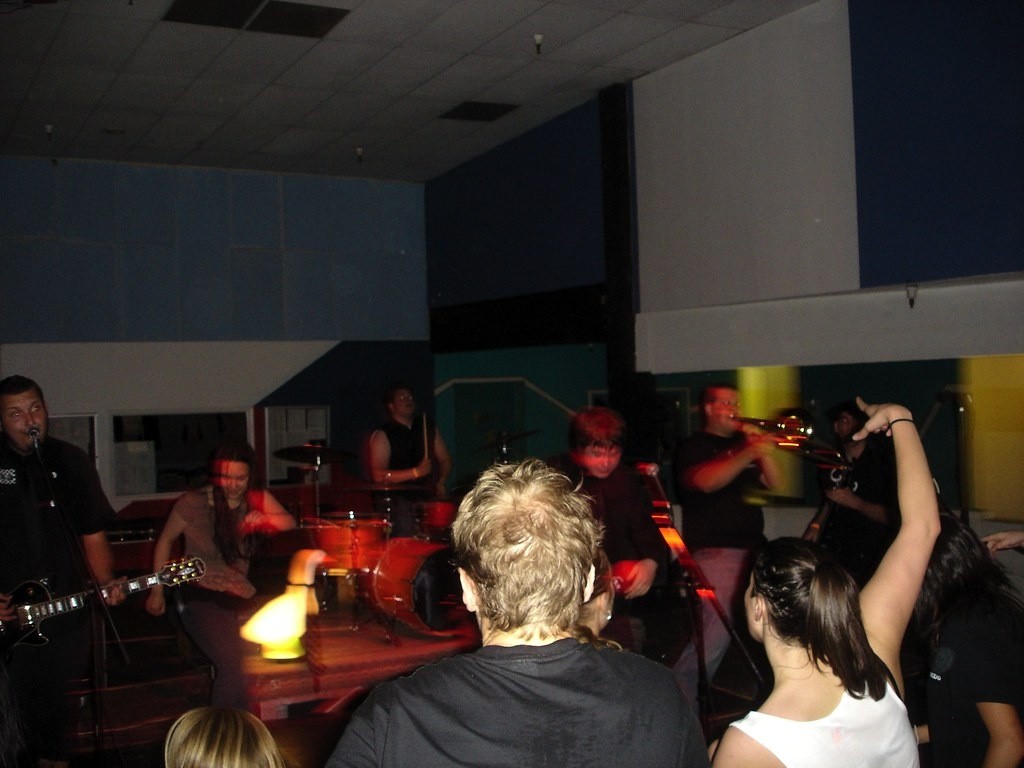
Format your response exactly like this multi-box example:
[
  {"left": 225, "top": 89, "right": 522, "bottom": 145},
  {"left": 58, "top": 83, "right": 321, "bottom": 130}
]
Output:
[
  {"left": 372, "top": 533, "right": 475, "bottom": 634},
  {"left": 317, "top": 512, "right": 390, "bottom": 579}
]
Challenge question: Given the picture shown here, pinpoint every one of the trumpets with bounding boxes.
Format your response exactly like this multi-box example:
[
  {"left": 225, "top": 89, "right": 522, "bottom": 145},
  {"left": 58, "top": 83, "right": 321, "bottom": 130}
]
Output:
[{"left": 730, "top": 408, "right": 816, "bottom": 443}]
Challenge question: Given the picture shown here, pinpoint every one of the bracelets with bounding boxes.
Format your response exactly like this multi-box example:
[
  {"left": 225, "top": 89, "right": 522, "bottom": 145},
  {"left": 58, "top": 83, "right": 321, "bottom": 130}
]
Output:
[
  {"left": 888, "top": 417, "right": 914, "bottom": 425},
  {"left": 413, "top": 467, "right": 418, "bottom": 477},
  {"left": 810, "top": 524, "right": 820, "bottom": 528},
  {"left": 914, "top": 724, "right": 919, "bottom": 745}
]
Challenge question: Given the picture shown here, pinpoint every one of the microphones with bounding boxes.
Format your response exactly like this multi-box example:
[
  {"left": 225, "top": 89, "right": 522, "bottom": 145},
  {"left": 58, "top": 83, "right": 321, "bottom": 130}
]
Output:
[
  {"left": 27, "top": 424, "right": 41, "bottom": 447},
  {"left": 636, "top": 463, "right": 660, "bottom": 476}
]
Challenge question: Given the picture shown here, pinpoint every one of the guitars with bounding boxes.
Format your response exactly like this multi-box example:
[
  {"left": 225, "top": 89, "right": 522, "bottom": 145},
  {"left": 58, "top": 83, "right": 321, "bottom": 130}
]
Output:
[
  {"left": 0, "top": 553, "right": 205, "bottom": 650},
  {"left": 804, "top": 465, "right": 866, "bottom": 547}
]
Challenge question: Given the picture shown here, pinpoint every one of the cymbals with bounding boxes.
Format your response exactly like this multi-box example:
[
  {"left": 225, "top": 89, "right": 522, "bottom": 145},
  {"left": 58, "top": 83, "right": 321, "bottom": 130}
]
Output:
[
  {"left": 339, "top": 483, "right": 427, "bottom": 493},
  {"left": 270, "top": 443, "right": 363, "bottom": 464}
]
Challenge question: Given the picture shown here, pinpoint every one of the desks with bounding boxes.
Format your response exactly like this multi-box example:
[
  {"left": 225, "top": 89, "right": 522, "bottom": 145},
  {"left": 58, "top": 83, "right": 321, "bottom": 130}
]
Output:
[{"left": 180, "top": 586, "right": 478, "bottom": 723}]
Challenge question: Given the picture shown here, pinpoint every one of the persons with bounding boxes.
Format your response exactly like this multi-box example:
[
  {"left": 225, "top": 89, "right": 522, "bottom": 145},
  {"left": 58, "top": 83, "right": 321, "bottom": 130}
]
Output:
[
  {"left": 370, "top": 387, "right": 452, "bottom": 538},
  {"left": 802, "top": 404, "right": 1024, "bottom": 768},
  {"left": 556, "top": 404, "right": 730, "bottom": 707},
  {"left": 673, "top": 384, "right": 775, "bottom": 548},
  {"left": 319, "top": 456, "right": 709, "bottom": 768},
  {"left": 145, "top": 436, "right": 299, "bottom": 709},
  {"left": 709, "top": 396, "right": 943, "bottom": 768},
  {"left": 162, "top": 703, "right": 283, "bottom": 768},
  {"left": 0, "top": 371, "right": 130, "bottom": 768}
]
